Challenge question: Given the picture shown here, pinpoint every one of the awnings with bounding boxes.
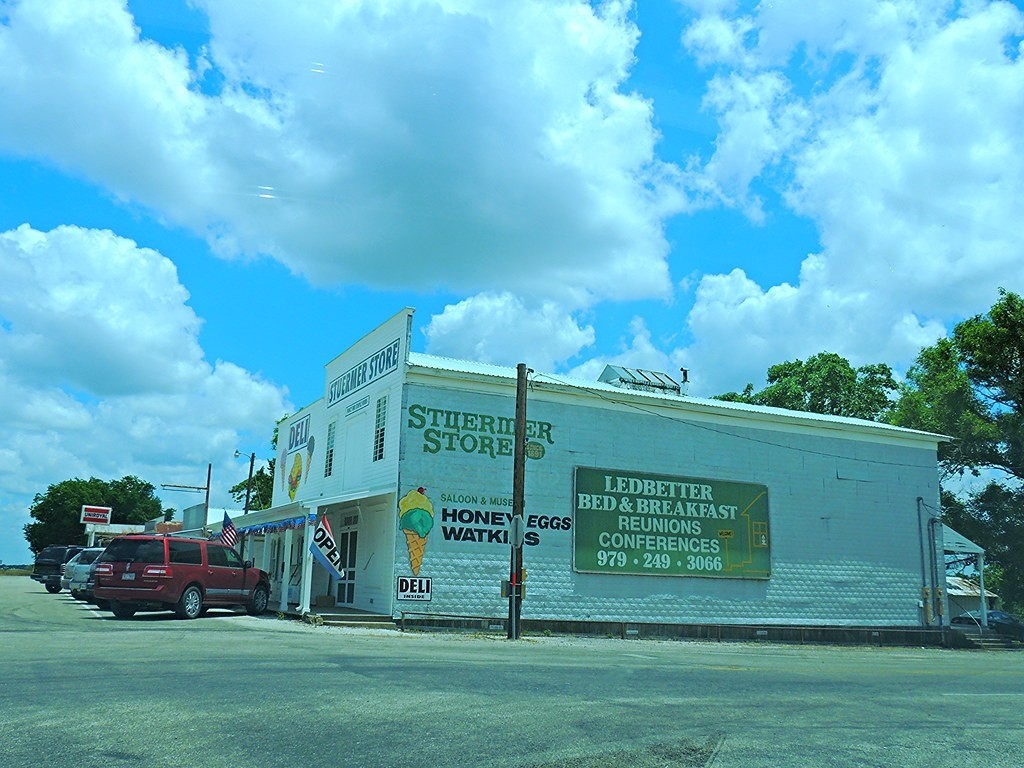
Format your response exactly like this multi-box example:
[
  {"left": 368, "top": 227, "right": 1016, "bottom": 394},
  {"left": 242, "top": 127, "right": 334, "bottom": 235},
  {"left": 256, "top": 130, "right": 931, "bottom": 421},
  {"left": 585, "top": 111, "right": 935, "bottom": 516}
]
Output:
[{"left": 947, "top": 576, "right": 1000, "bottom": 598}]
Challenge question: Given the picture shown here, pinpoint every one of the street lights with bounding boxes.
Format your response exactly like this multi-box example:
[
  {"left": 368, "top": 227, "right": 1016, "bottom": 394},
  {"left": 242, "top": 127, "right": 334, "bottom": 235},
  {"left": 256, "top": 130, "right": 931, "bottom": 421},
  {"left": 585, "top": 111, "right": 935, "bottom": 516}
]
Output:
[{"left": 234, "top": 450, "right": 255, "bottom": 558}]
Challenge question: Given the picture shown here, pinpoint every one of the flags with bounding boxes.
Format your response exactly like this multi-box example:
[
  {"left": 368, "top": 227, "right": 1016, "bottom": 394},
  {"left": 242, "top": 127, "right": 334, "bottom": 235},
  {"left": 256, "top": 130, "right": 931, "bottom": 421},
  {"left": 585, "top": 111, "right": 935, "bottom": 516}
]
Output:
[
  {"left": 309, "top": 513, "right": 344, "bottom": 581},
  {"left": 220, "top": 511, "right": 239, "bottom": 547}
]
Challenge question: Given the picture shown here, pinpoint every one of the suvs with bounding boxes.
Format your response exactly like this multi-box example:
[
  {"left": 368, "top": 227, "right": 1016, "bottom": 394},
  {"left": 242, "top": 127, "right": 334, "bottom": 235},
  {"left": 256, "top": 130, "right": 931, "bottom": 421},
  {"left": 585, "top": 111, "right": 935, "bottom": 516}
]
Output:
[
  {"left": 30, "top": 544, "right": 95, "bottom": 593},
  {"left": 92, "top": 533, "right": 271, "bottom": 619}
]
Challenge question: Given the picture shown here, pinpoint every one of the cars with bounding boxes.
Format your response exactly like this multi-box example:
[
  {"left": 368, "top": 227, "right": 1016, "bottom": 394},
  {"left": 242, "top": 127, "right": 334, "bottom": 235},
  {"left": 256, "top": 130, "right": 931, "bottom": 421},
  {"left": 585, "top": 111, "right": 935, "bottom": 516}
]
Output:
[
  {"left": 950, "top": 611, "right": 1024, "bottom": 643},
  {"left": 69, "top": 548, "right": 107, "bottom": 604},
  {"left": 61, "top": 551, "right": 86, "bottom": 600}
]
